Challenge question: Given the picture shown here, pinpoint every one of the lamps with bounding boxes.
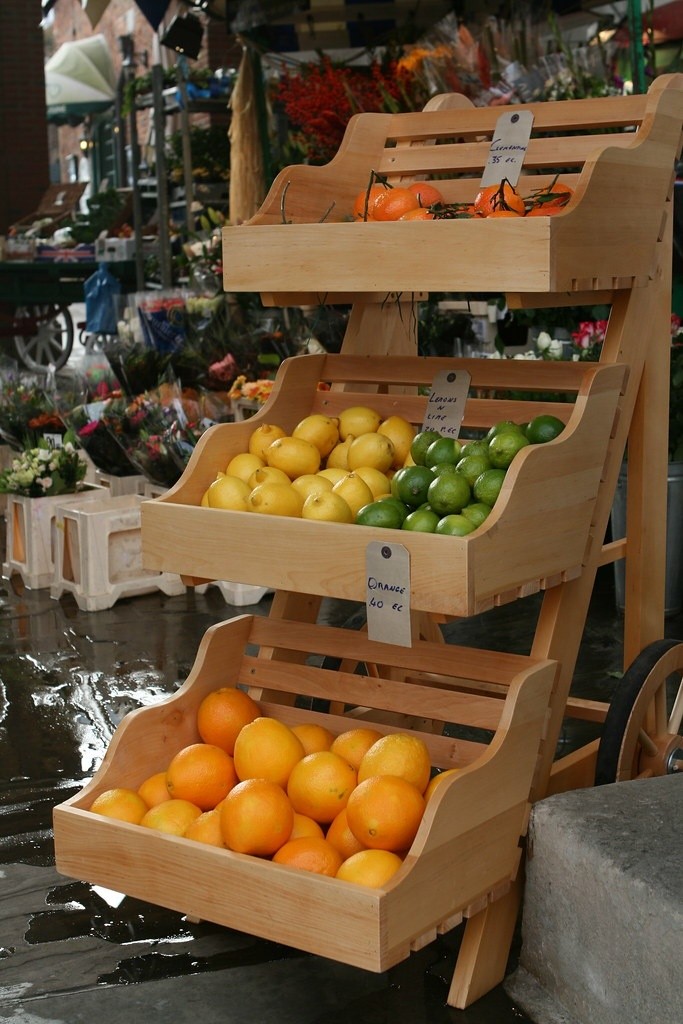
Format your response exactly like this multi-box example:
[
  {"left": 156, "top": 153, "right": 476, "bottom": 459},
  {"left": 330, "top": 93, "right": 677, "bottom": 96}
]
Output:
[{"left": 79, "top": 130, "right": 93, "bottom": 158}]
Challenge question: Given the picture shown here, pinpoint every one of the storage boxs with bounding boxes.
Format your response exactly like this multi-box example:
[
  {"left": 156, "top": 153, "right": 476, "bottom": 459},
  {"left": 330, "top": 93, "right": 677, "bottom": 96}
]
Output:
[
  {"left": 95, "top": 236, "right": 160, "bottom": 263},
  {"left": 9, "top": 181, "right": 89, "bottom": 239},
  {"left": 33, "top": 246, "right": 95, "bottom": 262}
]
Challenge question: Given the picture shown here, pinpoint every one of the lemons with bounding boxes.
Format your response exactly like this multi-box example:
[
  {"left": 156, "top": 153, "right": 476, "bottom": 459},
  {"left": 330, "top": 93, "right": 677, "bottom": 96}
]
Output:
[{"left": 197, "top": 406, "right": 564, "bottom": 536}]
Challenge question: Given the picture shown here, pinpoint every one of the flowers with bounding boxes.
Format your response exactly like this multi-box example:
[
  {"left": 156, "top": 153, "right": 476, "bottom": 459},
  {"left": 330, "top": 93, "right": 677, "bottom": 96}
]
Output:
[{"left": 0, "top": 0, "right": 683, "bottom": 494}]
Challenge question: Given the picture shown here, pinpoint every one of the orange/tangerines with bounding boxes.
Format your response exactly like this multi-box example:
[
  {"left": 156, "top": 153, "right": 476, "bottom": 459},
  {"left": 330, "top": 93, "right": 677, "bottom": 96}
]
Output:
[
  {"left": 348, "top": 182, "right": 574, "bottom": 223},
  {"left": 87, "top": 686, "right": 466, "bottom": 888}
]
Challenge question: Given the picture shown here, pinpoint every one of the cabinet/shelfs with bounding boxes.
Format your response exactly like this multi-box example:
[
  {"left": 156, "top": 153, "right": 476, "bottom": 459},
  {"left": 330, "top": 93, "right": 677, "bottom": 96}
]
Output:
[
  {"left": 123, "top": 65, "right": 232, "bottom": 294},
  {"left": 54, "top": 75, "right": 683, "bottom": 1011}
]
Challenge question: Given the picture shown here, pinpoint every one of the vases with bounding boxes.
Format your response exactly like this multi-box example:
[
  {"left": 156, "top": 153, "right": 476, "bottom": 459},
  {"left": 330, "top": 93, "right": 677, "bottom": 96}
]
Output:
[
  {"left": 0, "top": 448, "right": 275, "bottom": 611},
  {"left": 609, "top": 459, "right": 683, "bottom": 617}
]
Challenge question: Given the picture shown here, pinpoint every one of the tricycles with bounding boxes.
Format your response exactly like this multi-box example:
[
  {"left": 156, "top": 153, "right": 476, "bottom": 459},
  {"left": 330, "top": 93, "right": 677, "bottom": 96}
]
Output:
[{"left": 0, "top": 233, "right": 178, "bottom": 373}]
